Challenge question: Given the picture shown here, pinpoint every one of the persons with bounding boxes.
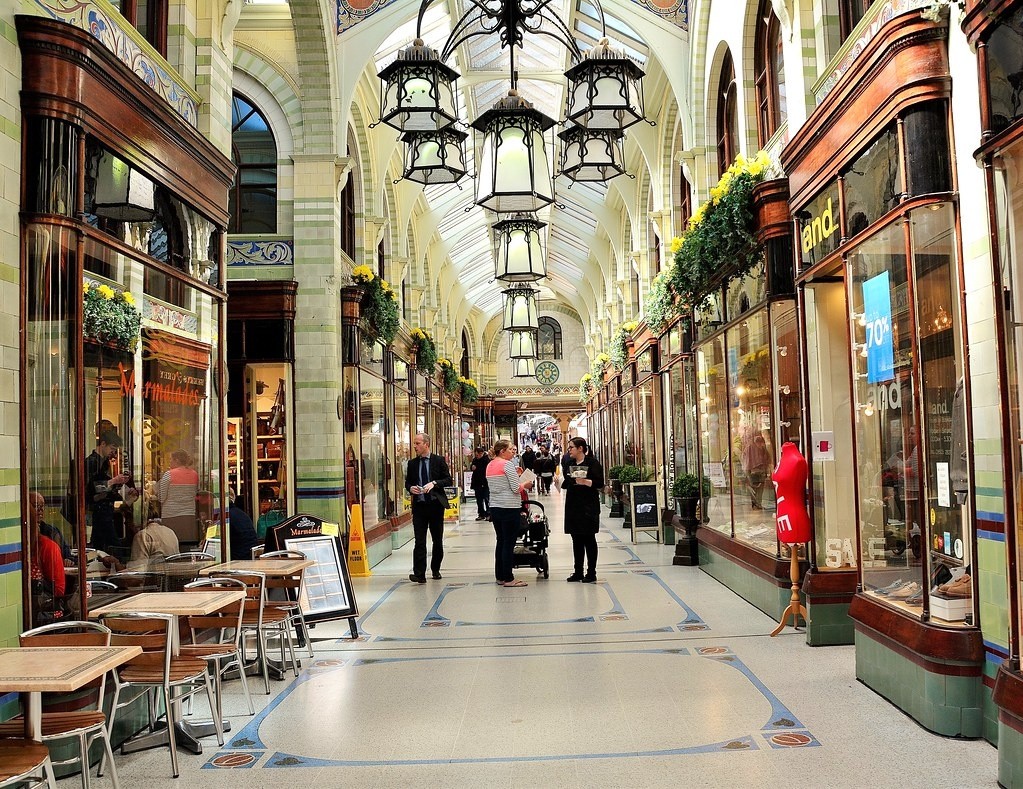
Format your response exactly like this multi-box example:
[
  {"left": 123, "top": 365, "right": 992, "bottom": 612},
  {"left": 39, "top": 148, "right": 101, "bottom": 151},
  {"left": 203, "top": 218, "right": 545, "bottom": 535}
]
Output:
[
  {"left": 29, "top": 419, "right": 256, "bottom": 615},
  {"left": 561, "top": 437, "right": 605, "bottom": 583},
  {"left": 405, "top": 433, "right": 453, "bottom": 583},
  {"left": 157, "top": 447, "right": 198, "bottom": 544},
  {"left": 771, "top": 442, "right": 812, "bottom": 544},
  {"left": 470, "top": 426, "right": 562, "bottom": 521},
  {"left": 485, "top": 439, "right": 534, "bottom": 587},
  {"left": 736, "top": 412, "right": 774, "bottom": 510}
]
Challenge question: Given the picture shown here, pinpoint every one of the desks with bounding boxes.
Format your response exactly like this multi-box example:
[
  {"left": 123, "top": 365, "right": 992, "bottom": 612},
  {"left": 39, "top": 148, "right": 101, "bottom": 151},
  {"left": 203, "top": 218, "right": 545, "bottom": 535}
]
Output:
[
  {"left": 199, "top": 560, "right": 317, "bottom": 681},
  {"left": 0, "top": 645, "right": 144, "bottom": 789},
  {"left": 89, "top": 591, "right": 247, "bottom": 755}
]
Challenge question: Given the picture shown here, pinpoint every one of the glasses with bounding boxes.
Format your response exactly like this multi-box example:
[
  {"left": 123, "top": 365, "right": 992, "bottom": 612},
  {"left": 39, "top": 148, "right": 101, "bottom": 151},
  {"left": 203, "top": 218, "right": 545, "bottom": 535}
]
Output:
[{"left": 567, "top": 446, "right": 574, "bottom": 451}]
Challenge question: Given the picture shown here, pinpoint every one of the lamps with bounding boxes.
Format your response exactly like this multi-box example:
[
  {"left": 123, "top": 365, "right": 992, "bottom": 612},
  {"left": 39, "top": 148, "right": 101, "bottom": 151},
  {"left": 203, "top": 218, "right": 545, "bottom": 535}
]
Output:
[
  {"left": 369, "top": 0, "right": 659, "bottom": 379},
  {"left": 777, "top": 346, "right": 792, "bottom": 428},
  {"left": 92, "top": 136, "right": 157, "bottom": 222},
  {"left": 849, "top": 311, "right": 874, "bottom": 421}
]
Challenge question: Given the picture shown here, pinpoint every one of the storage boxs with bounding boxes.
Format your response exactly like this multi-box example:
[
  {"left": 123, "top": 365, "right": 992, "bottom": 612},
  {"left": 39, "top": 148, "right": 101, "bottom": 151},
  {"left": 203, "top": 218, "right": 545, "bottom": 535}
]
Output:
[{"left": 929, "top": 594, "right": 972, "bottom": 622}]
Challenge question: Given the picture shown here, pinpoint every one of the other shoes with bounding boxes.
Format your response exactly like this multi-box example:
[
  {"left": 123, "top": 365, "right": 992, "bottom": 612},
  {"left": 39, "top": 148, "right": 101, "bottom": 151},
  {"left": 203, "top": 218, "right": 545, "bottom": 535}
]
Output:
[
  {"left": 566, "top": 568, "right": 585, "bottom": 582},
  {"left": 475, "top": 516, "right": 485, "bottom": 521},
  {"left": 582, "top": 569, "right": 597, "bottom": 583},
  {"left": 432, "top": 572, "right": 442, "bottom": 579},
  {"left": 409, "top": 574, "right": 427, "bottom": 584}
]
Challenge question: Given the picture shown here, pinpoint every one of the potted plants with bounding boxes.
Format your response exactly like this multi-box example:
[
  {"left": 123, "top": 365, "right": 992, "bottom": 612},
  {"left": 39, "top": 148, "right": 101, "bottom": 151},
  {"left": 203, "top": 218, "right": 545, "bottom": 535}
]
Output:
[
  {"left": 608, "top": 465, "right": 701, "bottom": 566},
  {"left": 702, "top": 477, "right": 714, "bottom": 525}
]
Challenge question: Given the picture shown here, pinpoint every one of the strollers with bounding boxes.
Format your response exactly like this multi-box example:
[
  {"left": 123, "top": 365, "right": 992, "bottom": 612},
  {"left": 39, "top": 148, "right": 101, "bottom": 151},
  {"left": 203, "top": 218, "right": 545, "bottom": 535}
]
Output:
[
  {"left": 872, "top": 468, "right": 921, "bottom": 559},
  {"left": 513, "top": 500, "right": 549, "bottom": 579},
  {"left": 196, "top": 490, "right": 220, "bottom": 535}
]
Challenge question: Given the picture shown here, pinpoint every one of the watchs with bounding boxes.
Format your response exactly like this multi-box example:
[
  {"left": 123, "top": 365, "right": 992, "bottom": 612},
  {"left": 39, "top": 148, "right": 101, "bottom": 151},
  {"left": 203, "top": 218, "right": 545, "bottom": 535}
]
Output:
[{"left": 432, "top": 481, "right": 437, "bottom": 488}]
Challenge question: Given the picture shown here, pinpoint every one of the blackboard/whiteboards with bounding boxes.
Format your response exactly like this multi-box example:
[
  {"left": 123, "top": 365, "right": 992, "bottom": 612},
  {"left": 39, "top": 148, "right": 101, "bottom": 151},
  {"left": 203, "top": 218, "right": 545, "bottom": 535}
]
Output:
[{"left": 630, "top": 482, "right": 663, "bottom": 531}]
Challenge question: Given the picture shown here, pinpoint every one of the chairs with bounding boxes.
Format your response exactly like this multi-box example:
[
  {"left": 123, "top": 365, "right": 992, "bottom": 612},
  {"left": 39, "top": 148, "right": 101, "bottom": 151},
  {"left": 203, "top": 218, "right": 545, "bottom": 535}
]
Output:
[{"left": 0, "top": 550, "right": 317, "bottom": 789}]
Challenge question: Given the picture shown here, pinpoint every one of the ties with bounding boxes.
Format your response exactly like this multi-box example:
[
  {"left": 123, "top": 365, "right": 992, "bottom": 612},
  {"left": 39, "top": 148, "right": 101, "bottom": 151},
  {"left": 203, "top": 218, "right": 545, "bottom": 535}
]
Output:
[{"left": 421, "top": 457, "right": 433, "bottom": 504}]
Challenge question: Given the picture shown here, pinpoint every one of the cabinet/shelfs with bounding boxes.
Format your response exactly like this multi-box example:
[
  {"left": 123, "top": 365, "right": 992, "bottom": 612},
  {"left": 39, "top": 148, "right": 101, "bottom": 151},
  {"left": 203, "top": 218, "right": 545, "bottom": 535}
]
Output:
[{"left": 228, "top": 362, "right": 295, "bottom": 526}]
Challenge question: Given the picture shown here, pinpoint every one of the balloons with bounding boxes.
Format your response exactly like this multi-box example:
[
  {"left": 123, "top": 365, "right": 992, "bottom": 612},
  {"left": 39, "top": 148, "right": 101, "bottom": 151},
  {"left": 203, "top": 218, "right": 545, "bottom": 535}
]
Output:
[
  {"left": 461, "top": 439, "right": 471, "bottom": 447},
  {"left": 462, "top": 422, "right": 470, "bottom": 431},
  {"left": 462, "top": 431, "right": 469, "bottom": 439},
  {"left": 462, "top": 447, "right": 471, "bottom": 455}
]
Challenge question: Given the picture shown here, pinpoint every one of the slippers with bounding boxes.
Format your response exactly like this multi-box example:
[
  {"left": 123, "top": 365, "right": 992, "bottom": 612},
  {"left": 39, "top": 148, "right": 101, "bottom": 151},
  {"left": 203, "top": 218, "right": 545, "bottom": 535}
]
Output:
[{"left": 496, "top": 580, "right": 528, "bottom": 587}]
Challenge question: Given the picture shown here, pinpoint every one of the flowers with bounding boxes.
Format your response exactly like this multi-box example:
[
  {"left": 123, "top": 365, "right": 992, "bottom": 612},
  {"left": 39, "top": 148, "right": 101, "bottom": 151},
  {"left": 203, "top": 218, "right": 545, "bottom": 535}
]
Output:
[
  {"left": 610, "top": 321, "right": 639, "bottom": 373},
  {"left": 83, "top": 282, "right": 142, "bottom": 355},
  {"left": 644, "top": 151, "right": 774, "bottom": 332},
  {"left": 351, "top": 265, "right": 480, "bottom": 407},
  {"left": 696, "top": 344, "right": 771, "bottom": 385},
  {"left": 579, "top": 374, "right": 592, "bottom": 403},
  {"left": 592, "top": 353, "right": 610, "bottom": 393}
]
